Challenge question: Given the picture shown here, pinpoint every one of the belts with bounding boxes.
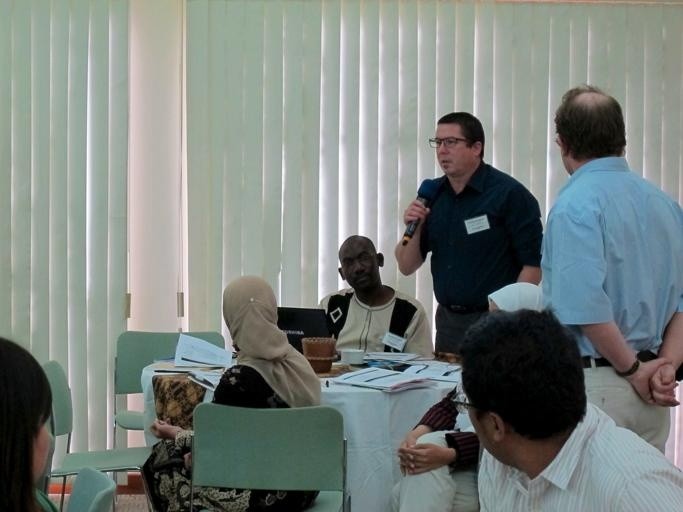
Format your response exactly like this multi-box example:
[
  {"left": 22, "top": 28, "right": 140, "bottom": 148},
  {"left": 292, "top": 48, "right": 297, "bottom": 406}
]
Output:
[{"left": 584, "top": 352, "right": 659, "bottom": 366}]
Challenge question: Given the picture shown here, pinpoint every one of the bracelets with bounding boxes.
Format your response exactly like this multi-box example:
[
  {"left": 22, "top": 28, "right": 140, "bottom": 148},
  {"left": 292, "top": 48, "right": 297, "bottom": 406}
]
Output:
[{"left": 614, "top": 358, "right": 640, "bottom": 377}]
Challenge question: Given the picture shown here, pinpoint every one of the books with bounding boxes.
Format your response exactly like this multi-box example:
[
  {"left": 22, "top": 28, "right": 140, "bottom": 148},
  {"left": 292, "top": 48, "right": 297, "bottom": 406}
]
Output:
[{"left": 153, "top": 332, "right": 462, "bottom": 395}]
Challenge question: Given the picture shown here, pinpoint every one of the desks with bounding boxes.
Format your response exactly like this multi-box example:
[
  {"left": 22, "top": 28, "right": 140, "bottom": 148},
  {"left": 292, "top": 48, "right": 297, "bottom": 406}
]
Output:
[{"left": 323, "top": 356, "right": 456, "bottom": 512}]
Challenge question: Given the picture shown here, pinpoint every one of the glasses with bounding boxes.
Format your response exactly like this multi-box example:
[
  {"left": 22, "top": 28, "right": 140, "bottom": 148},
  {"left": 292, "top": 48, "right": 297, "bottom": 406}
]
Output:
[
  {"left": 450, "top": 391, "right": 478, "bottom": 417},
  {"left": 428, "top": 135, "right": 470, "bottom": 149}
]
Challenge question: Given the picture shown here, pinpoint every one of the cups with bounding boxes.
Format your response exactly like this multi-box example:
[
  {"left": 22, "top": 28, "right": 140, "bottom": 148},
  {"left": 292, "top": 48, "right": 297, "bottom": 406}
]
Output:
[{"left": 301, "top": 338, "right": 336, "bottom": 373}]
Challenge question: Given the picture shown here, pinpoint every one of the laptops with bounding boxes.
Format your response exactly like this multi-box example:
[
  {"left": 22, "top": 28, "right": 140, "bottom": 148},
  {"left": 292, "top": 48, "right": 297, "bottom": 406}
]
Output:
[{"left": 276, "top": 307, "right": 329, "bottom": 355}]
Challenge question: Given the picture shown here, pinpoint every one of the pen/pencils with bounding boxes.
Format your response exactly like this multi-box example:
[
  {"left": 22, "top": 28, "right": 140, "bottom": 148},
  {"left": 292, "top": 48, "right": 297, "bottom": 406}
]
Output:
[{"left": 326, "top": 381, "right": 329, "bottom": 387}]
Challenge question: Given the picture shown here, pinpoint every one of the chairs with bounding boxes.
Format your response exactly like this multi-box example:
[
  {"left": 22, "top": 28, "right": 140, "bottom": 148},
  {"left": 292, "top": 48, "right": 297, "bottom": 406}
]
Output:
[
  {"left": 67, "top": 466, "right": 117, "bottom": 510},
  {"left": 41, "top": 360, "right": 151, "bottom": 510},
  {"left": 190, "top": 400, "right": 352, "bottom": 512},
  {"left": 111, "top": 330, "right": 225, "bottom": 510}
]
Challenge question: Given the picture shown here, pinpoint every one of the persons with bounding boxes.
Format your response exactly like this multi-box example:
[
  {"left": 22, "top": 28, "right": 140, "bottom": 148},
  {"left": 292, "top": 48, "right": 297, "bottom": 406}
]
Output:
[
  {"left": 394, "top": 111, "right": 543, "bottom": 358},
  {"left": 316, "top": 234, "right": 433, "bottom": 357},
  {"left": 390, "top": 279, "right": 548, "bottom": 512},
  {"left": 142, "top": 273, "right": 326, "bottom": 512},
  {"left": 538, "top": 81, "right": 683, "bottom": 459},
  {"left": 460, "top": 306, "right": 683, "bottom": 512},
  {"left": 0, "top": 335, "right": 61, "bottom": 512}
]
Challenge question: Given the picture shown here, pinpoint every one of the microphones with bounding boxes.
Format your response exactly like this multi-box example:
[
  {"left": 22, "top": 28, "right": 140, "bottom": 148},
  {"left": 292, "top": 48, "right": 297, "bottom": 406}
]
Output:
[{"left": 402, "top": 179, "right": 436, "bottom": 246}]
